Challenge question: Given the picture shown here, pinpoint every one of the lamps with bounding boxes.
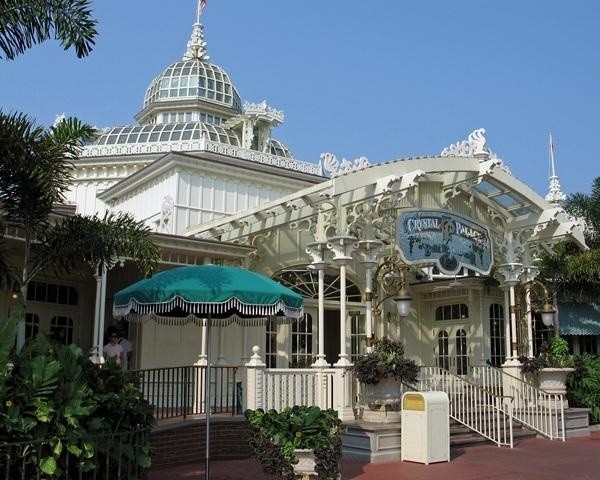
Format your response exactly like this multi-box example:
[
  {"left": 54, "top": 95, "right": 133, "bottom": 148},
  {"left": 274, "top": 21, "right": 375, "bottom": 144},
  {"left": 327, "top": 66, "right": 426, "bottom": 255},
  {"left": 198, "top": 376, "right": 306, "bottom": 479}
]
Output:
[
  {"left": 370, "top": 259, "right": 412, "bottom": 319},
  {"left": 517, "top": 277, "right": 556, "bottom": 327}
]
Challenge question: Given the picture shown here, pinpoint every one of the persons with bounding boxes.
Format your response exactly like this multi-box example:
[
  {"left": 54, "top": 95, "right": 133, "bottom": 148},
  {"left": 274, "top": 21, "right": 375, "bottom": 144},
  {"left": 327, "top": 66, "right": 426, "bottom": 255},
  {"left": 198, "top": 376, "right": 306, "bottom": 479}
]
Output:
[
  {"left": 103, "top": 330, "right": 126, "bottom": 371},
  {"left": 117, "top": 330, "right": 133, "bottom": 370}
]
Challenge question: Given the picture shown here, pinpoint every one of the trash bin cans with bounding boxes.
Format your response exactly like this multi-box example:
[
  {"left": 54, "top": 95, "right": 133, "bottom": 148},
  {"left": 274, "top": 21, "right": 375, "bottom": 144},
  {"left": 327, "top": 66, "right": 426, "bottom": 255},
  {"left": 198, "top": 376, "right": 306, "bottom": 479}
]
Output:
[{"left": 401, "top": 391, "right": 450, "bottom": 465}]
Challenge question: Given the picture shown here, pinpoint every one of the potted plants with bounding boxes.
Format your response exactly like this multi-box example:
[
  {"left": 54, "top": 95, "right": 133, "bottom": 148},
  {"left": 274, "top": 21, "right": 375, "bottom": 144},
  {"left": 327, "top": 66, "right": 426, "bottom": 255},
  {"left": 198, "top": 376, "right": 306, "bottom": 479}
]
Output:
[
  {"left": 242, "top": 405, "right": 348, "bottom": 480},
  {"left": 522, "top": 336, "right": 579, "bottom": 396},
  {"left": 351, "top": 338, "right": 422, "bottom": 409}
]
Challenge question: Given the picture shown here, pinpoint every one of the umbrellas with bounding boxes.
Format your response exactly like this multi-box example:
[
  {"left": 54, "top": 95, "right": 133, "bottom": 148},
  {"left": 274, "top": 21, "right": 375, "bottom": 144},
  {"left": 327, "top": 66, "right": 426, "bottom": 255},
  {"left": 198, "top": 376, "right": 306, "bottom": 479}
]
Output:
[{"left": 113, "top": 263, "right": 308, "bottom": 354}]
